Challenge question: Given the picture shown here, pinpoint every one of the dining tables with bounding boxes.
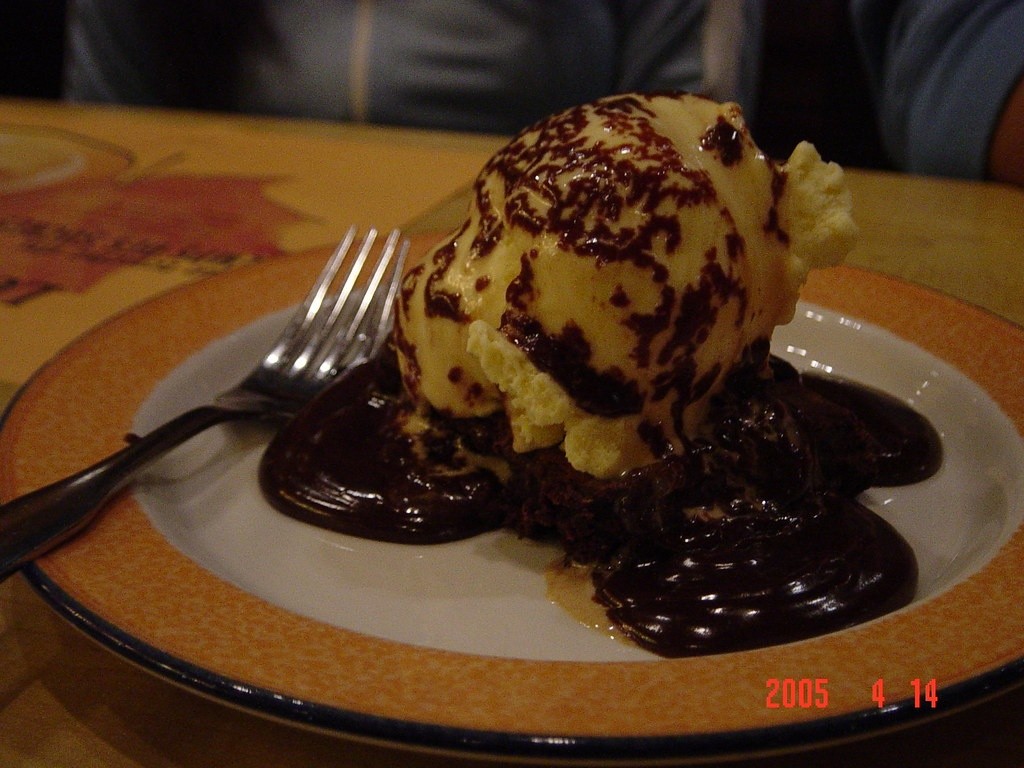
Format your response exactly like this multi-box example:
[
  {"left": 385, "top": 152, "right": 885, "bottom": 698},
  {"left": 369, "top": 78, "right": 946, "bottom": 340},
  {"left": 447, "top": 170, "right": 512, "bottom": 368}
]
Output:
[{"left": 0, "top": 99, "right": 1024, "bottom": 768}]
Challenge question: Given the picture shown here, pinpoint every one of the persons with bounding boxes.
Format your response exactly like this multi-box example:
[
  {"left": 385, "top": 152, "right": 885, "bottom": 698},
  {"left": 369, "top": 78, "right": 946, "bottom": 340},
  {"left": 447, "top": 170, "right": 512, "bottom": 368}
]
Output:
[{"left": 55, "top": 1, "right": 1024, "bottom": 186}]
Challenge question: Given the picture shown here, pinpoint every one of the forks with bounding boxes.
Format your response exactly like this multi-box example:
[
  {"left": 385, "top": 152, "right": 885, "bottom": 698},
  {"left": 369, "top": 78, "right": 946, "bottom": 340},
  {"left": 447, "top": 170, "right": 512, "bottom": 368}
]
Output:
[{"left": 0, "top": 224, "right": 409, "bottom": 585}]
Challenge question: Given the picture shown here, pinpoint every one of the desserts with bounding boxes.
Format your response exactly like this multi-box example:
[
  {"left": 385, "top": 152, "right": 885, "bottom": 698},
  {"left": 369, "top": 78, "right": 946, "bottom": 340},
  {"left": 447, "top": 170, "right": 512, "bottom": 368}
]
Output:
[{"left": 257, "top": 85, "right": 944, "bottom": 660}]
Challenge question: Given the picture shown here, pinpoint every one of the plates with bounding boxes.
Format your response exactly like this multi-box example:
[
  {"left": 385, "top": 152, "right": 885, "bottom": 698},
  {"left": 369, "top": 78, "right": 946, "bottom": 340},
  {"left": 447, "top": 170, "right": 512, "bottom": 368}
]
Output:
[{"left": 1, "top": 230, "right": 1023, "bottom": 766}]
